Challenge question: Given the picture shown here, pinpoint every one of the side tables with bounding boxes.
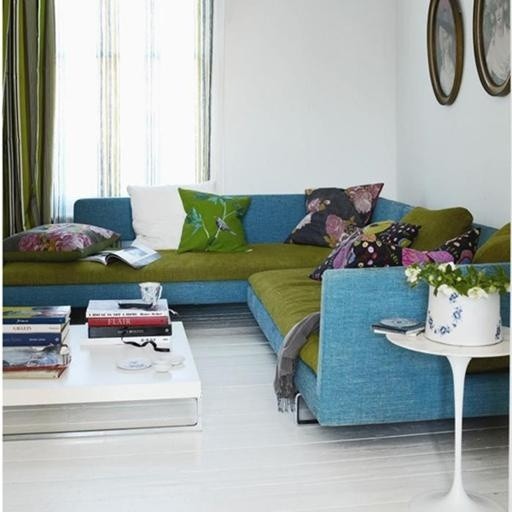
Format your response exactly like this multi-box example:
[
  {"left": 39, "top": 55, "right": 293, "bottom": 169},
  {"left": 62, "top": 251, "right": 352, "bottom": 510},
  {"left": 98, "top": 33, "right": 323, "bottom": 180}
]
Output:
[{"left": 386, "top": 322, "right": 510, "bottom": 512}]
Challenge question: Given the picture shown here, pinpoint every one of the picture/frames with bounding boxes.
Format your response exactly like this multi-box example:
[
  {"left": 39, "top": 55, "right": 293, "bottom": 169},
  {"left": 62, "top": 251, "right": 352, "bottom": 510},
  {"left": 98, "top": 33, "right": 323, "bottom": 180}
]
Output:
[{"left": 427, "top": 1, "right": 510, "bottom": 106}]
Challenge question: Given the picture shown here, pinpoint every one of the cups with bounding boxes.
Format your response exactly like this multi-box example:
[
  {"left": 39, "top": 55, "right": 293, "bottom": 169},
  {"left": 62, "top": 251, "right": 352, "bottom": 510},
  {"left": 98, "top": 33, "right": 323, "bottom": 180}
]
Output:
[{"left": 139, "top": 281, "right": 163, "bottom": 306}]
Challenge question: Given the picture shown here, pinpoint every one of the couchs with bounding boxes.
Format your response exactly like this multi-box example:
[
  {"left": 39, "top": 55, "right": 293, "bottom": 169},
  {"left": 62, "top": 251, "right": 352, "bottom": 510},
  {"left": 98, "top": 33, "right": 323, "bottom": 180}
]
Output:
[
  {"left": 3, "top": 194, "right": 404, "bottom": 308},
  {"left": 247, "top": 206, "right": 509, "bottom": 424}
]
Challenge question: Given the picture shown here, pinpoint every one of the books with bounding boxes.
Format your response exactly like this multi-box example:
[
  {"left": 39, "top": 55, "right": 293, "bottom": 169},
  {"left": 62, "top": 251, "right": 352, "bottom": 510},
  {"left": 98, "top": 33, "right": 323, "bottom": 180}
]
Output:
[
  {"left": 3, "top": 305, "right": 70, "bottom": 379},
  {"left": 77, "top": 243, "right": 160, "bottom": 269},
  {"left": 85, "top": 299, "right": 173, "bottom": 348},
  {"left": 372, "top": 316, "right": 425, "bottom": 337}
]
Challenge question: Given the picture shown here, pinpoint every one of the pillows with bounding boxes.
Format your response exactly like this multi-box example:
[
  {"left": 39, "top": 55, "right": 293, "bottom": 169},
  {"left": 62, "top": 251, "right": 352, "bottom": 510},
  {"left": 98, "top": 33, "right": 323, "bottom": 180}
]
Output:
[
  {"left": 283, "top": 183, "right": 384, "bottom": 248},
  {"left": 401, "top": 226, "right": 484, "bottom": 266},
  {"left": 128, "top": 180, "right": 253, "bottom": 254},
  {"left": 5, "top": 222, "right": 122, "bottom": 263},
  {"left": 306, "top": 223, "right": 421, "bottom": 284}
]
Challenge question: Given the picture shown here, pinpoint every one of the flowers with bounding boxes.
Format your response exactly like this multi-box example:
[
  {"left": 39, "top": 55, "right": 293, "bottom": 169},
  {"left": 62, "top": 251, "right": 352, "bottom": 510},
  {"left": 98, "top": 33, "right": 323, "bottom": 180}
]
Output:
[{"left": 405, "top": 261, "right": 511, "bottom": 300}]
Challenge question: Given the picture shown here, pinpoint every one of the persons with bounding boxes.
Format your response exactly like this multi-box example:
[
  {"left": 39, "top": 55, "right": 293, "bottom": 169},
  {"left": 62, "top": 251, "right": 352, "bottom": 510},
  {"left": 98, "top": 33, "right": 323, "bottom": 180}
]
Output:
[
  {"left": 486, "top": 0, "right": 510, "bottom": 83},
  {"left": 438, "top": 18, "right": 455, "bottom": 92}
]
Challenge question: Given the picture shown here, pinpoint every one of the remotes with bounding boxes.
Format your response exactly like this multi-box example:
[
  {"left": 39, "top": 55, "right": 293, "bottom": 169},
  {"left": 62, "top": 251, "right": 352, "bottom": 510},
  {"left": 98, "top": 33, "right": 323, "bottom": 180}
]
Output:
[{"left": 118, "top": 299, "right": 153, "bottom": 308}]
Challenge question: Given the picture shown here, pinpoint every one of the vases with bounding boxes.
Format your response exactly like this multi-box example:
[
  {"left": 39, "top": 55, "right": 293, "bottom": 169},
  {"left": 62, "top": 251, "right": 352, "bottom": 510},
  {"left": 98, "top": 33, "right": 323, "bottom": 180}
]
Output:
[{"left": 424, "top": 284, "right": 502, "bottom": 346}]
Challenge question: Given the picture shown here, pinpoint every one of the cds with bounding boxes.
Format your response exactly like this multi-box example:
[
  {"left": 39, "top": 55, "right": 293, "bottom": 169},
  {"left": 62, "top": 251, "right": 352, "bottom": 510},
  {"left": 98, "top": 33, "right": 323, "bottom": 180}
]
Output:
[{"left": 380, "top": 317, "right": 420, "bottom": 329}]
